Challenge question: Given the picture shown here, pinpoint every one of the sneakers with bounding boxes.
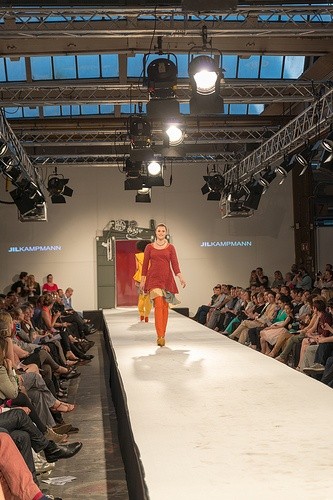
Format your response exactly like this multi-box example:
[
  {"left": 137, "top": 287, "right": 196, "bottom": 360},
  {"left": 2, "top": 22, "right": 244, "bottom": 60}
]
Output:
[{"left": 303, "top": 362, "right": 325, "bottom": 374}]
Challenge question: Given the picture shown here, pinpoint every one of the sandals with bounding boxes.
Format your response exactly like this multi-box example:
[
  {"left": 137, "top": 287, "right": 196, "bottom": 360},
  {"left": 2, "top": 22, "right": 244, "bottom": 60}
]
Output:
[{"left": 51, "top": 402, "right": 75, "bottom": 413}]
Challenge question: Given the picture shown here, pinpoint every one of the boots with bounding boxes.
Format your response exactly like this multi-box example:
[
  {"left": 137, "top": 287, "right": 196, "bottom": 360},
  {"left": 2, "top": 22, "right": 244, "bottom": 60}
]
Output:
[{"left": 44, "top": 426, "right": 67, "bottom": 444}]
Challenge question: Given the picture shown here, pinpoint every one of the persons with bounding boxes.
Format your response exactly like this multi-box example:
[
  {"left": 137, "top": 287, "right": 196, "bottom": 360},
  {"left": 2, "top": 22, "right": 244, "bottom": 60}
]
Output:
[
  {"left": 132, "top": 240, "right": 152, "bottom": 322},
  {"left": 193, "top": 264, "right": 333, "bottom": 388},
  {"left": 0, "top": 271, "right": 97, "bottom": 500},
  {"left": 140, "top": 224, "right": 186, "bottom": 347}
]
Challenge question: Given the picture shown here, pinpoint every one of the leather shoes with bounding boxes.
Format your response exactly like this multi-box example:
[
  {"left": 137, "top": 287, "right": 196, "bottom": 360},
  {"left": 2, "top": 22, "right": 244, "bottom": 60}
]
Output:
[{"left": 45, "top": 442, "right": 82, "bottom": 463}]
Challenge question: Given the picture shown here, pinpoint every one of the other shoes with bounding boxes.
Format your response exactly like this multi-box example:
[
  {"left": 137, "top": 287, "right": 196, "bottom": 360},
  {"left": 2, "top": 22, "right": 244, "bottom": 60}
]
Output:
[
  {"left": 54, "top": 354, "right": 79, "bottom": 375},
  {"left": 54, "top": 424, "right": 79, "bottom": 433},
  {"left": 157, "top": 337, "right": 165, "bottom": 347},
  {"left": 58, "top": 388, "right": 68, "bottom": 397},
  {"left": 39, "top": 494, "right": 62, "bottom": 500},
  {"left": 84, "top": 355, "right": 94, "bottom": 360},
  {"left": 34, "top": 459, "right": 55, "bottom": 474}
]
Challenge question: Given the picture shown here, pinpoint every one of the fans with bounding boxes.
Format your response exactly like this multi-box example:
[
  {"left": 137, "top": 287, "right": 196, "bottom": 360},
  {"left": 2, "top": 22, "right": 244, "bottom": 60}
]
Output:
[{"left": 201, "top": 164, "right": 230, "bottom": 201}]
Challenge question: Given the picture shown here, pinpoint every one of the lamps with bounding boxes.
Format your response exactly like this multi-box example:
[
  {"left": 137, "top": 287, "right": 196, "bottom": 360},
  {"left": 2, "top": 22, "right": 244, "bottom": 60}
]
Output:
[
  {"left": 0, "top": 139, "right": 47, "bottom": 222},
  {"left": 46, "top": 166, "right": 73, "bottom": 204},
  {"left": 116, "top": 38, "right": 187, "bottom": 204},
  {"left": 227, "top": 128, "right": 333, "bottom": 217},
  {"left": 187, "top": 27, "right": 225, "bottom": 116}
]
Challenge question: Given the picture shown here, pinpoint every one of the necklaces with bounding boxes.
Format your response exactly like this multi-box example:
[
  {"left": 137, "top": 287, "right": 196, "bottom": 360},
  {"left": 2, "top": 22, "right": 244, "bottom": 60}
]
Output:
[{"left": 156, "top": 241, "right": 166, "bottom": 246}]
